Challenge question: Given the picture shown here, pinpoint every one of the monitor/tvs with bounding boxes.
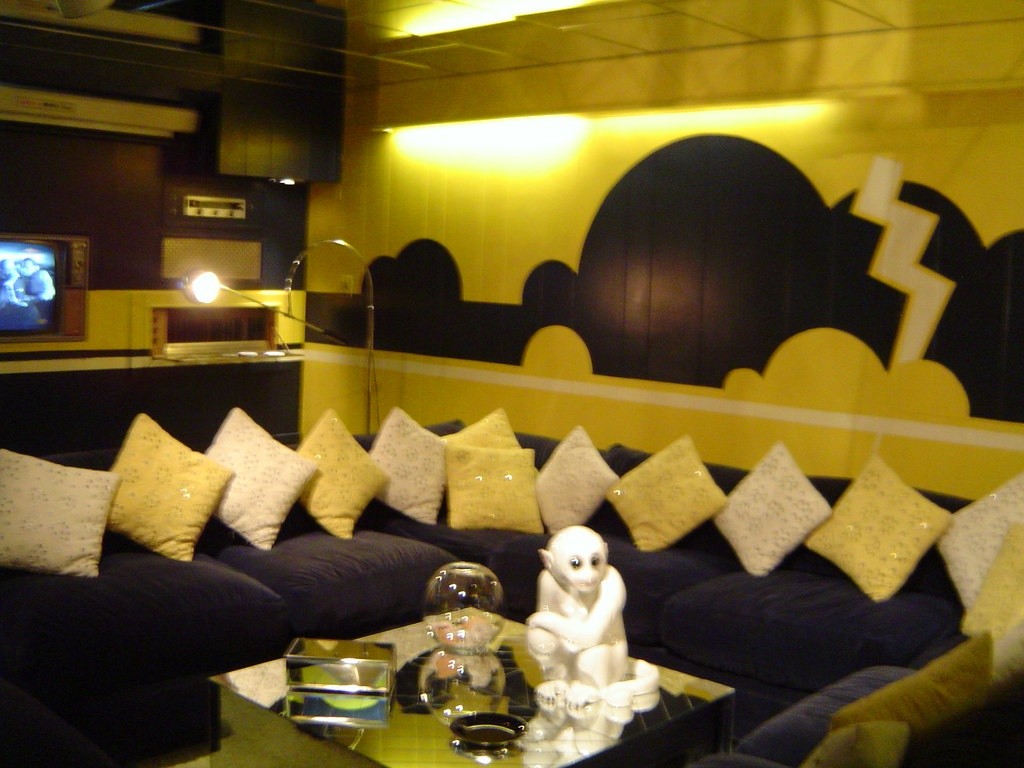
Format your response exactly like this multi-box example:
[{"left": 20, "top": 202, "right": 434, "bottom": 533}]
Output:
[{"left": 0, "top": 232, "right": 91, "bottom": 344}]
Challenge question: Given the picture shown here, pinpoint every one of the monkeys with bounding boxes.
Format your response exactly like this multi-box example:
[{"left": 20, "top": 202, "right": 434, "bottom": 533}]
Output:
[{"left": 525, "top": 524, "right": 659, "bottom": 709}]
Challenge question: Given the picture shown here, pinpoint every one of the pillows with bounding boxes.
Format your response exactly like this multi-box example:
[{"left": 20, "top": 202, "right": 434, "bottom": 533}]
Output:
[
  {"left": 535, "top": 425, "right": 619, "bottom": 533},
  {"left": 445, "top": 446, "right": 544, "bottom": 535},
  {"left": 714, "top": 443, "right": 832, "bottom": 577},
  {"left": 607, "top": 435, "right": 729, "bottom": 551},
  {"left": 804, "top": 454, "right": 951, "bottom": 603},
  {"left": 0, "top": 448, "right": 122, "bottom": 577},
  {"left": 440, "top": 407, "right": 539, "bottom": 476},
  {"left": 959, "top": 522, "right": 1024, "bottom": 638},
  {"left": 102, "top": 413, "right": 231, "bottom": 562},
  {"left": 936, "top": 470, "right": 1024, "bottom": 609},
  {"left": 204, "top": 407, "right": 317, "bottom": 552},
  {"left": 368, "top": 406, "right": 446, "bottom": 526},
  {"left": 295, "top": 409, "right": 387, "bottom": 539}
]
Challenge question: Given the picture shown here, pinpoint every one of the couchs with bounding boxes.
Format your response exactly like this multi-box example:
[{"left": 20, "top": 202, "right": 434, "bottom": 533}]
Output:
[{"left": 0, "top": 421, "right": 1024, "bottom": 768}]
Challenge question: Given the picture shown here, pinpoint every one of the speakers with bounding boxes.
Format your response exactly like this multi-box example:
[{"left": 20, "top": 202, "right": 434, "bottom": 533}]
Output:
[{"left": 207, "top": 98, "right": 341, "bottom": 185}]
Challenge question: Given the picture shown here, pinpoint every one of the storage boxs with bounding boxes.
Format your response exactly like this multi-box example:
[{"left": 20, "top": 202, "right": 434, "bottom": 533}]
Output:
[
  {"left": 284, "top": 638, "right": 396, "bottom": 691},
  {"left": 286, "top": 685, "right": 394, "bottom": 728}
]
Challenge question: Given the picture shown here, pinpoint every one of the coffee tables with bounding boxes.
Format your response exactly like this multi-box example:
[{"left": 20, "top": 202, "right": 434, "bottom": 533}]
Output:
[{"left": 206, "top": 607, "right": 735, "bottom": 768}]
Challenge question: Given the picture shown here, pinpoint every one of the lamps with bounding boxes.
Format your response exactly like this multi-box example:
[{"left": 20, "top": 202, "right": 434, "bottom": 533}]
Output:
[{"left": 179, "top": 239, "right": 373, "bottom": 435}]
